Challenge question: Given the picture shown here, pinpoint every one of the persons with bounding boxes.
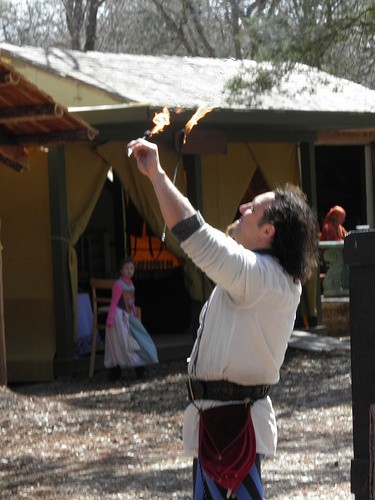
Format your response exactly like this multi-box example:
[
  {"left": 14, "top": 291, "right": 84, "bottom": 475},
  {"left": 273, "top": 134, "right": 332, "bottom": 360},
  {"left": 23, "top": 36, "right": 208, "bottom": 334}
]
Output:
[
  {"left": 127, "top": 138, "right": 319, "bottom": 499},
  {"left": 318, "top": 205, "right": 349, "bottom": 242},
  {"left": 104, "top": 257, "right": 158, "bottom": 384}
]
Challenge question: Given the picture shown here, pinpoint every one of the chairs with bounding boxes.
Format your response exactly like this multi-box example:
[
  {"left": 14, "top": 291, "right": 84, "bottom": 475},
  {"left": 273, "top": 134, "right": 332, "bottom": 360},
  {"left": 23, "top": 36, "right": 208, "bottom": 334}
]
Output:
[{"left": 87, "top": 277, "right": 141, "bottom": 377}]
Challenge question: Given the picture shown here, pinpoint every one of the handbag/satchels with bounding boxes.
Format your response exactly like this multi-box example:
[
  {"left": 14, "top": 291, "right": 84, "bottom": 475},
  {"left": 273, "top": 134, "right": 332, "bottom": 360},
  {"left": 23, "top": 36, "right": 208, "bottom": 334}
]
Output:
[{"left": 198, "top": 404, "right": 256, "bottom": 494}]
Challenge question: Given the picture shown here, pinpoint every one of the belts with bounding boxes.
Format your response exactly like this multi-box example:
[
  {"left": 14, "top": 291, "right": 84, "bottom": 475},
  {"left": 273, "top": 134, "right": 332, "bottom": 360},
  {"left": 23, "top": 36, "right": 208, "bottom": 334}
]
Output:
[{"left": 185, "top": 380, "right": 270, "bottom": 403}]
